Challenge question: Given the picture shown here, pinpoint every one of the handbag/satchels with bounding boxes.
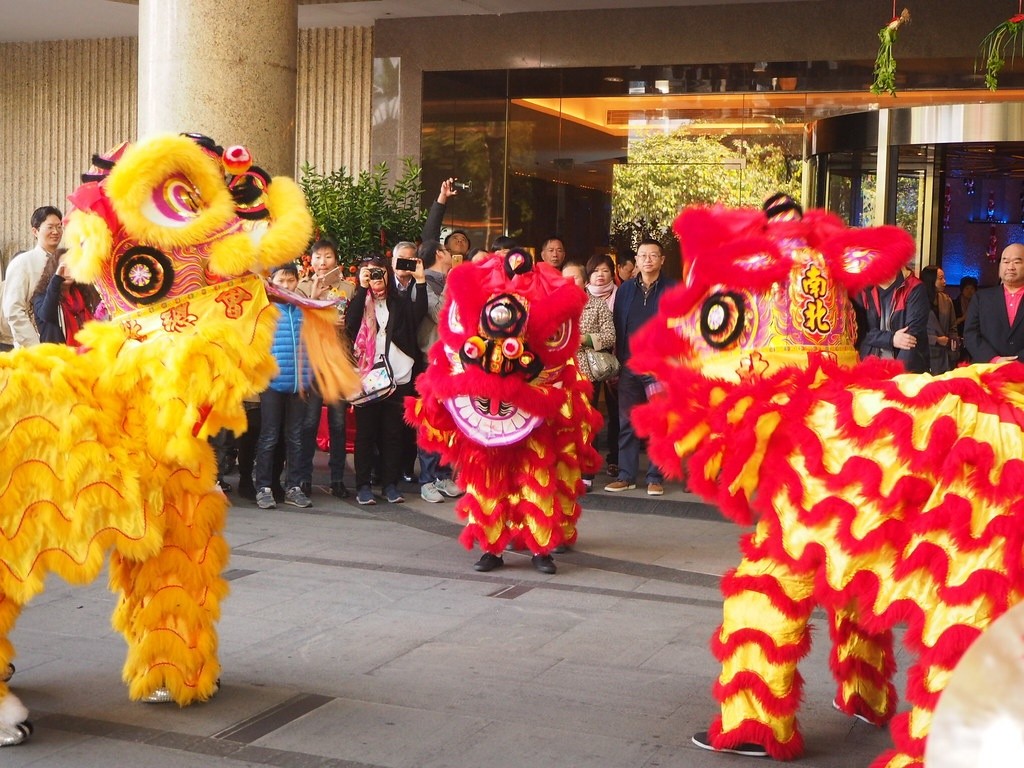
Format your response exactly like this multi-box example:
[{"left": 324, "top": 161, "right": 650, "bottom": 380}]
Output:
[
  {"left": 583, "top": 346, "right": 624, "bottom": 381},
  {"left": 345, "top": 353, "right": 396, "bottom": 408}
]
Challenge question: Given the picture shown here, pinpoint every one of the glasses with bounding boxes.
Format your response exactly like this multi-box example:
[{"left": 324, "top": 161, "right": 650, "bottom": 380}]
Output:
[
  {"left": 361, "top": 255, "right": 386, "bottom": 261},
  {"left": 38, "top": 226, "right": 65, "bottom": 233},
  {"left": 638, "top": 253, "right": 662, "bottom": 260}
]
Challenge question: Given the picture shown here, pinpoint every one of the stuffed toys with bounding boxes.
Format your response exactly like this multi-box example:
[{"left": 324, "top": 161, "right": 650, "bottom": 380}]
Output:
[
  {"left": 0, "top": 134, "right": 310, "bottom": 746},
  {"left": 629, "top": 202, "right": 1024, "bottom": 768},
  {"left": 404, "top": 251, "right": 605, "bottom": 572}
]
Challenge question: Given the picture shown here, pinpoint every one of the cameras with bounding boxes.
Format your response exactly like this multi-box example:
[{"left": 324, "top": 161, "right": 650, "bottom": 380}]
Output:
[{"left": 366, "top": 269, "right": 383, "bottom": 280}]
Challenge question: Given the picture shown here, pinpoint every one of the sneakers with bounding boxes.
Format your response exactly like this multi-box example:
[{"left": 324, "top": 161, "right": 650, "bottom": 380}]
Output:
[
  {"left": 646, "top": 481, "right": 664, "bottom": 494},
  {"left": 256, "top": 487, "right": 277, "bottom": 509},
  {"left": 531, "top": 555, "right": 557, "bottom": 573},
  {"left": 473, "top": 553, "right": 504, "bottom": 572},
  {"left": 603, "top": 479, "right": 636, "bottom": 491},
  {"left": 381, "top": 484, "right": 405, "bottom": 502},
  {"left": 420, "top": 482, "right": 446, "bottom": 503},
  {"left": 238, "top": 479, "right": 257, "bottom": 504},
  {"left": 285, "top": 488, "right": 313, "bottom": 507},
  {"left": 273, "top": 485, "right": 288, "bottom": 502},
  {"left": 435, "top": 478, "right": 462, "bottom": 497},
  {"left": 356, "top": 485, "right": 375, "bottom": 505}
]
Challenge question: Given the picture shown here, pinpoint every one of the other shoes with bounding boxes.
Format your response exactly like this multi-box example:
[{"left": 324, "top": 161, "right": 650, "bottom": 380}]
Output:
[
  {"left": 217, "top": 477, "right": 232, "bottom": 493},
  {"left": 606, "top": 464, "right": 618, "bottom": 476},
  {"left": 329, "top": 481, "right": 348, "bottom": 496},
  {"left": 692, "top": 732, "right": 768, "bottom": 756},
  {"left": 551, "top": 544, "right": 567, "bottom": 554},
  {"left": 401, "top": 473, "right": 420, "bottom": 485},
  {"left": 833, "top": 698, "right": 875, "bottom": 725},
  {"left": 584, "top": 481, "right": 594, "bottom": 492}
]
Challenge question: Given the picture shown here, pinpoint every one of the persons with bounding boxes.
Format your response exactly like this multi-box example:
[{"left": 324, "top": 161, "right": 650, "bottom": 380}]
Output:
[
  {"left": 0, "top": 206, "right": 107, "bottom": 352},
  {"left": 207, "top": 237, "right": 696, "bottom": 509},
  {"left": 422, "top": 177, "right": 470, "bottom": 256},
  {"left": 963, "top": 242, "right": 1024, "bottom": 363},
  {"left": 850, "top": 240, "right": 977, "bottom": 374}
]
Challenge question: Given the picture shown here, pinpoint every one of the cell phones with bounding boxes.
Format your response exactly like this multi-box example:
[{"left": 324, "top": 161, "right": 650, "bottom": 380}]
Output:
[
  {"left": 452, "top": 255, "right": 463, "bottom": 268},
  {"left": 452, "top": 179, "right": 472, "bottom": 193},
  {"left": 320, "top": 267, "right": 344, "bottom": 287},
  {"left": 396, "top": 258, "right": 417, "bottom": 272},
  {"left": 63, "top": 267, "right": 71, "bottom": 278}
]
